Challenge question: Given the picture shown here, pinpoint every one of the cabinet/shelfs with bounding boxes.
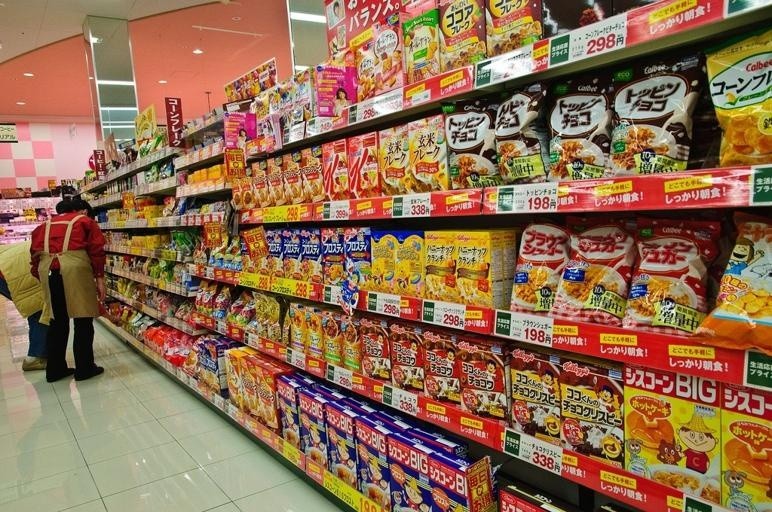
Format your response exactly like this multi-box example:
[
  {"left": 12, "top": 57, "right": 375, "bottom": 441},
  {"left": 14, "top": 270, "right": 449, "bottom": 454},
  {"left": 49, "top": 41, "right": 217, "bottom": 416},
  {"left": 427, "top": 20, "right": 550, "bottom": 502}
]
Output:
[
  {"left": 1, "top": 192, "right": 63, "bottom": 246},
  {"left": 75, "top": 0, "right": 770, "bottom": 512}
]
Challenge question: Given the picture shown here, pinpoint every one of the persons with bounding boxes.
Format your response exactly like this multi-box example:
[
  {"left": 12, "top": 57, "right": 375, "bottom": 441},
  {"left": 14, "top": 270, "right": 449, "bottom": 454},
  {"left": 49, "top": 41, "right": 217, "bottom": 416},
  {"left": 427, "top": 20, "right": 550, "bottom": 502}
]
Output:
[
  {"left": 0, "top": 241, "right": 48, "bottom": 371},
  {"left": 30, "top": 198, "right": 106, "bottom": 384},
  {"left": 236, "top": 128, "right": 247, "bottom": 147},
  {"left": 332, "top": 88, "right": 351, "bottom": 115}
]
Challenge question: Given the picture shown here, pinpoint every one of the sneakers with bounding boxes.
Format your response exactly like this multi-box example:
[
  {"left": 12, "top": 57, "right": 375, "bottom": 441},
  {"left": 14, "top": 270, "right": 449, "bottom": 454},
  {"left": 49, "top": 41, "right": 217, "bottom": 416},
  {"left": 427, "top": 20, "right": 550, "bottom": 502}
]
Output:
[
  {"left": 23, "top": 359, "right": 48, "bottom": 370},
  {"left": 74, "top": 367, "right": 104, "bottom": 381},
  {"left": 46, "top": 368, "right": 74, "bottom": 382}
]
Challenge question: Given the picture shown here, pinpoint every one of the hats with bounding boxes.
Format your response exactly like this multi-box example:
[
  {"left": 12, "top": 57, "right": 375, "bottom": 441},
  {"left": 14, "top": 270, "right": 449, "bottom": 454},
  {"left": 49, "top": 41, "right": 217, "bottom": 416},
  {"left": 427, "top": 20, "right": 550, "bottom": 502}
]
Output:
[
  {"left": 369, "top": 456, "right": 381, "bottom": 470},
  {"left": 406, "top": 477, "right": 422, "bottom": 494},
  {"left": 450, "top": 504, "right": 464, "bottom": 512},
  {"left": 285, "top": 406, "right": 292, "bottom": 415},
  {"left": 677, "top": 414, "right": 716, "bottom": 433},
  {"left": 338, "top": 438, "right": 348, "bottom": 451},
  {"left": 310, "top": 423, "right": 319, "bottom": 434}
]
